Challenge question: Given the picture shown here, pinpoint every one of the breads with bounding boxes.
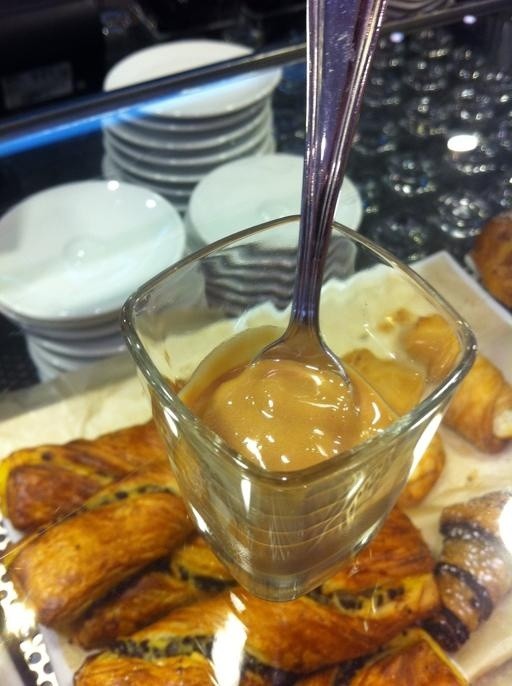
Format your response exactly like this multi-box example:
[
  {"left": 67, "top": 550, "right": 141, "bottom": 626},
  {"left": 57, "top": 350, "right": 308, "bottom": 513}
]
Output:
[
  {"left": 233, "top": 493, "right": 511, "bottom": 686},
  {"left": 410, "top": 316, "right": 511, "bottom": 454},
  {"left": 474, "top": 212, "right": 512, "bottom": 312},
  {"left": 346, "top": 346, "right": 441, "bottom": 512},
  {"left": 1, "top": 418, "right": 233, "bottom": 686}
]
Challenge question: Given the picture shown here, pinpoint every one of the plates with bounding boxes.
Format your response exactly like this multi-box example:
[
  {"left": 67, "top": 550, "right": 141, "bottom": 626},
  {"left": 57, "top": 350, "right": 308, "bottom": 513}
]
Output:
[
  {"left": 0, "top": 248, "right": 512, "bottom": 686},
  {"left": 95, "top": 37, "right": 286, "bottom": 218},
  {"left": 0, "top": 176, "right": 207, "bottom": 383},
  {"left": 192, "top": 149, "right": 364, "bottom": 324}
]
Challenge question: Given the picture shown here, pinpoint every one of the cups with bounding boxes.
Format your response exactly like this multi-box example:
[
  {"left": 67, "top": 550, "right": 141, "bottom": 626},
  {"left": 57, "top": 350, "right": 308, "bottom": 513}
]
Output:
[{"left": 119, "top": 212, "right": 476, "bottom": 603}]
